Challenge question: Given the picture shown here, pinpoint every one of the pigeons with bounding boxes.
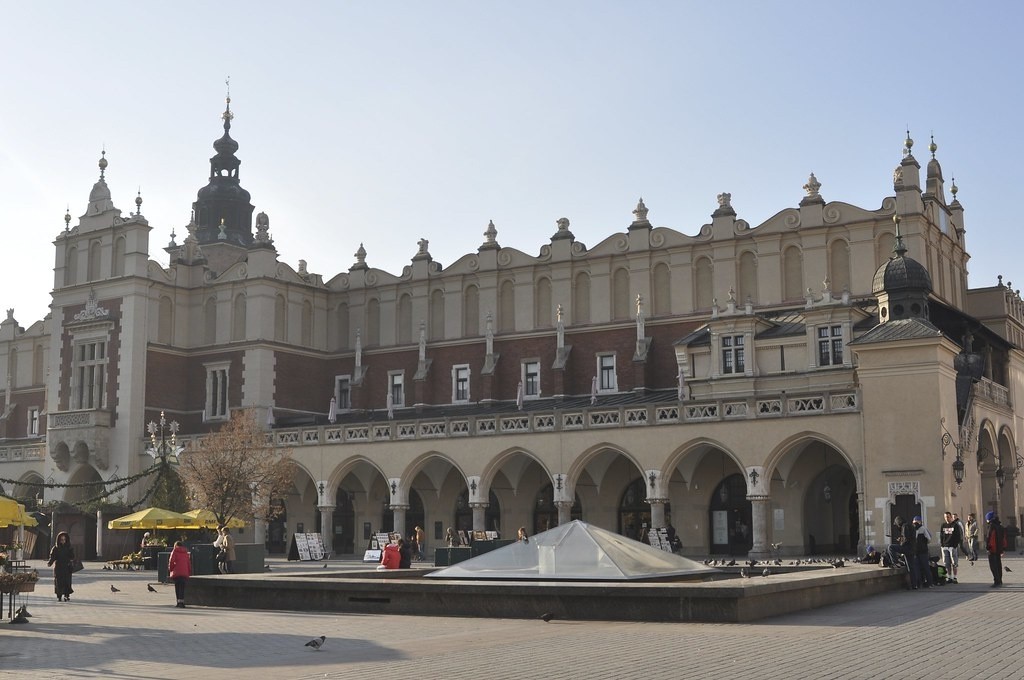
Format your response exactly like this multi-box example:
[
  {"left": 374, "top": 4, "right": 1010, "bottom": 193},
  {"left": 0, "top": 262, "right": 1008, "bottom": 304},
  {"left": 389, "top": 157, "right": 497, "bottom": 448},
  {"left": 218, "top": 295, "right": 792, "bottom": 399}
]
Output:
[
  {"left": 703, "top": 558, "right": 862, "bottom": 578},
  {"left": 147, "top": 584, "right": 157, "bottom": 592},
  {"left": 111, "top": 585, "right": 120, "bottom": 592},
  {"left": 540, "top": 613, "right": 553, "bottom": 622},
  {"left": 305, "top": 635, "right": 326, "bottom": 649},
  {"left": 1004, "top": 566, "right": 1012, "bottom": 572},
  {"left": 970, "top": 562, "right": 974, "bottom": 566}
]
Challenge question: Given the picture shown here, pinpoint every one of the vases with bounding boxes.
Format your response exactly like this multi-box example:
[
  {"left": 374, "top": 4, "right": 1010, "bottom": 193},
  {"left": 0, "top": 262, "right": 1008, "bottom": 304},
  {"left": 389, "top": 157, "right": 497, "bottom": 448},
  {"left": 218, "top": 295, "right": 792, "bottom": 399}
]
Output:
[
  {"left": 0, "top": 585, "right": 11, "bottom": 593},
  {"left": 19, "top": 582, "right": 35, "bottom": 592}
]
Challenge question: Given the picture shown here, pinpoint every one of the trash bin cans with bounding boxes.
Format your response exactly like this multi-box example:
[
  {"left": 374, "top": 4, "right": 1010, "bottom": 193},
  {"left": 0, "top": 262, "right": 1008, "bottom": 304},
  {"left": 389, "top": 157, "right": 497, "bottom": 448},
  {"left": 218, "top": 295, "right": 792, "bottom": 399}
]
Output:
[{"left": 157, "top": 552, "right": 174, "bottom": 583}]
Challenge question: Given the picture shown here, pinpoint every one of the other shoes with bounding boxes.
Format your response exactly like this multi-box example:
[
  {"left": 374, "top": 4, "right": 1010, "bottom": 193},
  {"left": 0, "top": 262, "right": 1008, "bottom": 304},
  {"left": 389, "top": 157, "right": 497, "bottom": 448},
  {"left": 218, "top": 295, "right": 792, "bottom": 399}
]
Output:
[
  {"left": 948, "top": 577, "right": 958, "bottom": 584},
  {"left": 929, "top": 584, "right": 934, "bottom": 588},
  {"left": 914, "top": 585, "right": 917, "bottom": 589},
  {"left": 175, "top": 601, "right": 185, "bottom": 608},
  {"left": 889, "top": 563, "right": 901, "bottom": 568},
  {"left": 990, "top": 584, "right": 1001, "bottom": 588},
  {"left": 969, "top": 554, "right": 977, "bottom": 561},
  {"left": 59, "top": 597, "right": 61, "bottom": 601},
  {"left": 64, "top": 596, "right": 70, "bottom": 602}
]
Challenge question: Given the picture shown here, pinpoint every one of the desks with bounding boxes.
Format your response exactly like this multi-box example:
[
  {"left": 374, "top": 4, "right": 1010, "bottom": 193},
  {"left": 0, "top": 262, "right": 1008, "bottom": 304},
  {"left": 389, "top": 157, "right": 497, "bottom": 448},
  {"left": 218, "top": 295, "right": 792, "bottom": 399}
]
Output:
[
  {"left": 0, "top": 560, "right": 32, "bottom": 624},
  {"left": 107, "top": 562, "right": 144, "bottom": 571}
]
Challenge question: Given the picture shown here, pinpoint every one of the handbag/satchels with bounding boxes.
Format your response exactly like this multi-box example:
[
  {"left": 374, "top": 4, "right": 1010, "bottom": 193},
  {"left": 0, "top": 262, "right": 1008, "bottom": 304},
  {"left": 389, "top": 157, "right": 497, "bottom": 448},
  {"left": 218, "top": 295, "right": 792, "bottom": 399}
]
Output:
[
  {"left": 933, "top": 565, "right": 948, "bottom": 583},
  {"left": 69, "top": 559, "right": 83, "bottom": 573},
  {"left": 216, "top": 551, "right": 228, "bottom": 562}
]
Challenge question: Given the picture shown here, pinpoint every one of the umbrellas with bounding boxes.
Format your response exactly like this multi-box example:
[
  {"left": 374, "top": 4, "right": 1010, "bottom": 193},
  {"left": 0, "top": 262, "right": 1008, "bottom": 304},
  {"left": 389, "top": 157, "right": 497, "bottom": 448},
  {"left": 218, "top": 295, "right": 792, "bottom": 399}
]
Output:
[
  {"left": 328, "top": 397, "right": 337, "bottom": 424},
  {"left": 387, "top": 393, "right": 395, "bottom": 420},
  {"left": 676, "top": 369, "right": 686, "bottom": 402},
  {"left": 108, "top": 507, "right": 188, "bottom": 536},
  {"left": 266, "top": 405, "right": 276, "bottom": 428},
  {"left": 589, "top": 376, "right": 599, "bottom": 405},
  {"left": 0, "top": 496, "right": 39, "bottom": 530},
  {"left": 182, "top": 507, "right": 245, "bottom": 529},
  {"left": 516, "top": 379, "right": 525, "bottom": 412}
]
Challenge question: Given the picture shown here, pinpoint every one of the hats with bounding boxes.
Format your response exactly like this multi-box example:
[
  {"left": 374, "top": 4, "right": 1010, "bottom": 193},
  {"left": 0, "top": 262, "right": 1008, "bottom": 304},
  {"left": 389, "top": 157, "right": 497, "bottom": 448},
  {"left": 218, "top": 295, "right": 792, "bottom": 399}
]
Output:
[
  {"left": 913, "top": 516, "right": 921, "bottom": 520},
  {"left": 867, "top": 545, "right": 874, "bottom": 554},
  {"left": 984, "top": 511, "right": 994, "bottom": 520}
]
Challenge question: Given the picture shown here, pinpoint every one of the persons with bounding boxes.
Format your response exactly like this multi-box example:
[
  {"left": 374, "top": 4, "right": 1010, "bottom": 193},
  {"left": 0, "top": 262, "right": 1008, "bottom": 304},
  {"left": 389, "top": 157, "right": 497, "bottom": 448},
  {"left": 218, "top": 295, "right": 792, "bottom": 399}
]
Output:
[
  {"left": 397, "top": 539, "right": 411, "bottom": 569},
  {"left": 912, "top": 515, "right": 935, "bottom": 589},
  {"left": 952, "top": 514, "right": 971, "bottom": 559},
  {"left": 861, "top": 545, "right": 881, "bottom": 564},
  {"left": 213, "top": 524, "right": 236, "bottom": 574},
  {"left": 142, "top": 532, "right": 151, "bottom": 547},
  {"left": 518, "top": 527, "right": 527, "bottom": 540},
  {"left": 47, "top": 530, "right": 84, "bottom": 603},
  {"left": 965, "top": 514, "right": 979, "bottom": 561},
  {"left": 888, "top": 516, "right": 914, "bottom": 576},
  {"left": 445, "top": 527, "right": 461, "bottom": 547},
  {"left": 377, "top": 543, "right": 401, "bottom": 569},
  {"left": 415, "top": 526, "right": 425, "bottom": 562},
  {"left": 168, "top": 541, "right": 192, "bottom": 608},
  {"left": 668, "top": 524, "right": 683, "bottom": 555},
  {"left": 985, "top": 511, "right": 1003, "bottom": 588},
  {"left": 940, "top": 512, "right": 960, "bottom": 584}
]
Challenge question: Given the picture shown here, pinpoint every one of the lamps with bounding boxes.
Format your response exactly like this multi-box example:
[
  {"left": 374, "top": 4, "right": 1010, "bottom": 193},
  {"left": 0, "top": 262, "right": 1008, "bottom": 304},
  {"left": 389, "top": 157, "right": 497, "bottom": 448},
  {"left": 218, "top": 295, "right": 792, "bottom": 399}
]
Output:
[
  {"left": 649, "top": 471, "right": 656, "bottom": 489},
  {"left": 108, "top": 464, "right": 119, "bottom": 481},
  {"left": 941, "top": 417, "right": 965, "bottom": 486},
  {"left": 319, "top": 483, "right": 324, "bottom": 495},
  {"left": 470, "top": 479, "right": 477, "bottom": 496},
  {"left": 312, "top": 500, "right": 319, "bottom": 512},
  {"left": 749, "top": 469, "right": 760, "bottom": 488},
  {"left": 537, "top": 465, "right": 546, "bottom": 507},
  {"left": 719, "top": 451, "right": 728, "bottom": 502},
  {"left": 457, "top": 474, "right": 465, "bottom": 510},
  {"left": 390, "top": 481, "right": 397, "bottom": 495},
  {"left": 823, "top": 444, "right": 833, "bottom": 503},
  {"left": 44, "top": 468, "right": 55, "bottom": 488},
  {"left": 556, "top": 474, "right": 563, "bottom": 492},
  {"left": 976, "top": 448, "right": 1007, "bottom": 488},
  {"left": 626, "top": 460, "right": 635, "bottom": 506}
]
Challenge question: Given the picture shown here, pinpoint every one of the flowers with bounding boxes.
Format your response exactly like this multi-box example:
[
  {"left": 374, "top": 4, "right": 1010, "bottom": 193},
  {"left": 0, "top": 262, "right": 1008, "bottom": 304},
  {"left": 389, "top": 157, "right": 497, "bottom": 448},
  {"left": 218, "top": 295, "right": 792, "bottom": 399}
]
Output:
[{"left": 0, "top": 568, "right": 40, "bottom": 595}]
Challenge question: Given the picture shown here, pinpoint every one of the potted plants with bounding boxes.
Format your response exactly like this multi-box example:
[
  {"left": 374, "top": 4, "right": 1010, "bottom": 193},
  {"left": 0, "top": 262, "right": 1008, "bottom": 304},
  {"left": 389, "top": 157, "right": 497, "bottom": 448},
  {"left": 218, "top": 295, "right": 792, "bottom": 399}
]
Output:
[
  {"left": 124, "top": 550, "right": 142, "bottom": 562},
  {"left": 0, "top": 543, "right": 23, "bottom": 560},
  {"left": 140, "top": 533, "right": 169, "bottom": 549}
]
